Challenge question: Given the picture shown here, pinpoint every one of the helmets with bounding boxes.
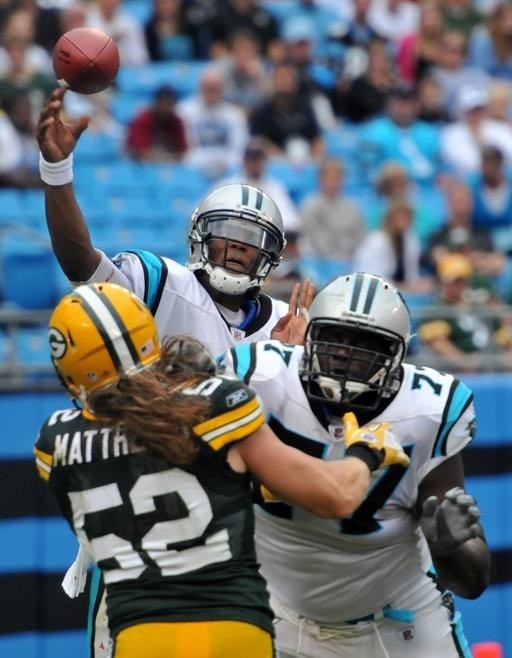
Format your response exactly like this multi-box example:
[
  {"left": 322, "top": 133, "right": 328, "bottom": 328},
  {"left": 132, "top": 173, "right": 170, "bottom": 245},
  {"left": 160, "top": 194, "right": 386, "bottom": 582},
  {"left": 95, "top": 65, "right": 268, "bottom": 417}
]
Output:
[
  {"left": 47, "top": 281, "right": 162, "bottom": 402},
  {"left": 300, "top": 271, "right": 412, "bottom": 405},
  {"left": 186, "top": 184, "right": 288, "bottom": 295}
]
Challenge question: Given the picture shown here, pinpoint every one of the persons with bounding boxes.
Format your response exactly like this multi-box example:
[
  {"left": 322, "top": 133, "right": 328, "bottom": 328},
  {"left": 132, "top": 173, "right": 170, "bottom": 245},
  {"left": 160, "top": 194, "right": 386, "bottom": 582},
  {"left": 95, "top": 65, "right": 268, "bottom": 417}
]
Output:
[
  {"left": 203, "top": 269, "right": 496, "bottom": 658},
  {"left": 36, "top": 84, "right": 320, "bottom": 658},
  {"left": 232, "top": 0, "right": 512, "bottom": 371},
  {"left": 29, "top": 280, "right": 409, "bottom": 657},
  {"left": 0, "top": 0, "right": 282, "bottom": 193}
]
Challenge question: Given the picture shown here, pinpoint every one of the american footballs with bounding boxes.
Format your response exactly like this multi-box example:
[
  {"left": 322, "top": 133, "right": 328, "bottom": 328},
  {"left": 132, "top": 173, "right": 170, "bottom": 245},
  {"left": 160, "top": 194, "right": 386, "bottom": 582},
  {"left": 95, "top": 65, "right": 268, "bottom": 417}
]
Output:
[{"left": 54, "top": 27, "right": 120, "bottom": 94}]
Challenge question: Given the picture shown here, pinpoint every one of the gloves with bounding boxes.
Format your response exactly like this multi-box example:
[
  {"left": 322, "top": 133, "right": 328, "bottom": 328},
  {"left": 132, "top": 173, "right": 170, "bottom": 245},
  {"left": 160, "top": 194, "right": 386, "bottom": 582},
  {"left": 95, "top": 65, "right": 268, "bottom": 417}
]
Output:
[
  {"left": 342, "top": 411, "right": 411, "bottom": 474},
  {"left": 419, "top": 486, "right": 483, "bottom": 561}
]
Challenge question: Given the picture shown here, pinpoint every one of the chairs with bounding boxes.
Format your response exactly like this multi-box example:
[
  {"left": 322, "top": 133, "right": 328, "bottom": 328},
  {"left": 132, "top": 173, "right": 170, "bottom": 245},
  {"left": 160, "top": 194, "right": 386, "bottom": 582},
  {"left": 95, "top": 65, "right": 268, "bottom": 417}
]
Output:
[{"left": 0, "top": 141, "right": 212, "bottom": 302}]
